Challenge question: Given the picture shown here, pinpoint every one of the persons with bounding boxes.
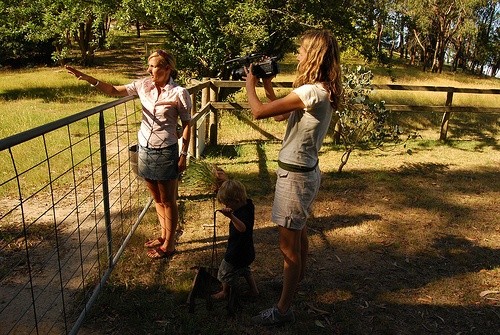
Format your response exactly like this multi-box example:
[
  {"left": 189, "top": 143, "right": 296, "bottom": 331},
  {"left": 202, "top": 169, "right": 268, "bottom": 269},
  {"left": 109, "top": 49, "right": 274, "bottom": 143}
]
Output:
[
  {"left": 242, "top": 29, "right": 344, "bottom": 328},
  {"left": 213, "top": 179, "right": 260, "bottom": 300},
  {"left": 65, "top": 50, "right": 191, "bottom": 259}
]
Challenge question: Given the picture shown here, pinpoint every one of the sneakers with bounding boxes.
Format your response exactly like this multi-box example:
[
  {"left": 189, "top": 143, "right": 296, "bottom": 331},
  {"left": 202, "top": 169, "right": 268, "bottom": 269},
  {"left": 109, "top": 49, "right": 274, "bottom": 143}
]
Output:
[
  {"left": 265, "top": 275, "right": 284, "bottom": 290},
  {"left": 252, "top": 304, "right": 294, "bottom": 327}
]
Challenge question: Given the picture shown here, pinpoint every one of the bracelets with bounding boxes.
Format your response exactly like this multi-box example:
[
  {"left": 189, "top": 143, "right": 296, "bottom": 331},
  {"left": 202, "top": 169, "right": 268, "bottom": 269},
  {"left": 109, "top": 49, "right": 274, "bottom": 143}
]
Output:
[
  {"left": 180, "top": 151, "right": 188, "bottom": 155},
  {"left": 91, "top": 80, "right": 100, "bottom": 87}
]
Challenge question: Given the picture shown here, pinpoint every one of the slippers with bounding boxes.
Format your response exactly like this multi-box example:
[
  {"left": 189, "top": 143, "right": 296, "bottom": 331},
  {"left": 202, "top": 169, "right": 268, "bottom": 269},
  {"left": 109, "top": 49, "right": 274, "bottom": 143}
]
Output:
[
  {"left": 147, "top": 248, "right": 177, "bottom": 260},
  {"left": 144, "top": 237, "right": 166, "bottom": 249}
]
